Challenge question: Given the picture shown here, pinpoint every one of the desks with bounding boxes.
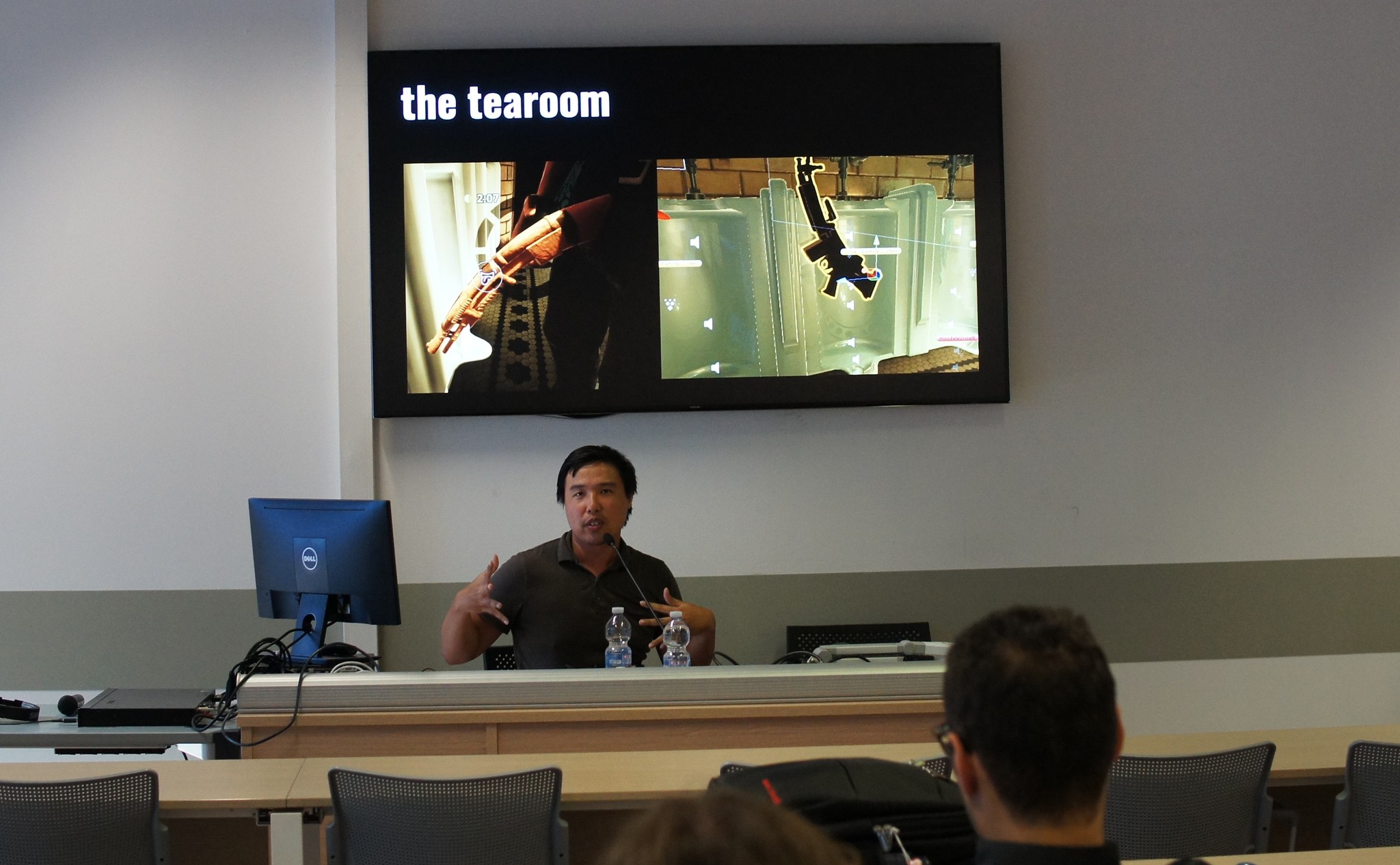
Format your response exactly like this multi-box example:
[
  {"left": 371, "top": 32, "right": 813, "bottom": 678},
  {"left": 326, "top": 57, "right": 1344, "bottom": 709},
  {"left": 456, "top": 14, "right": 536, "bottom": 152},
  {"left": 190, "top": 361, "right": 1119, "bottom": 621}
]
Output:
[
  {"left": 1, "top": 691, "right": 241, "bottom": 758},
  {"left": 0, "top": 722, "right": 1399, "bottom": 865},
  {"left": 235, "top": 702, "right": 949, "bottom": 758}
]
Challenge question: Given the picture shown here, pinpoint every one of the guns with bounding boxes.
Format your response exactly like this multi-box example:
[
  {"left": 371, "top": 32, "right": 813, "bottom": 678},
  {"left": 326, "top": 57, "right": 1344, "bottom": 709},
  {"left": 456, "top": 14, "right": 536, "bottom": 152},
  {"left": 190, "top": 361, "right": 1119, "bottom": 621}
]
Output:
[{"left": 794, "top": 155, "right": 881, "bottom": 301}]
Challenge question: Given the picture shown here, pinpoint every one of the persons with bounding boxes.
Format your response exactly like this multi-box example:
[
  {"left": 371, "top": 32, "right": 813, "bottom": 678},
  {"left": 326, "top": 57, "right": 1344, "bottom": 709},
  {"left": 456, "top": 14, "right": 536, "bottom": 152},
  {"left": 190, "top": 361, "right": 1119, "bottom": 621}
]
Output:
[
  {"left": 442, "top": 446, "right": 717, "bottom": 670},
  {"left": 931, "top": 602, "right": 1210, "bottom": 865},
  {"left": 584, "top": 790, "right": 862, "bottom": 865}
]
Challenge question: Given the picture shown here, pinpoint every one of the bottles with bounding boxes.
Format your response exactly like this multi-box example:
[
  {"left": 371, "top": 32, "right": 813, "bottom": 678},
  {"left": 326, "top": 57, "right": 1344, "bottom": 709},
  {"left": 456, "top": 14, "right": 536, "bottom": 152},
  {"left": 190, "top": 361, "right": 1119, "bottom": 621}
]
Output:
[
  {"left": 662, "top": 611, "right": 691, "bottom": 668},
  {"left": 605, "top": 607, "right": 632, "bottom": 669}
]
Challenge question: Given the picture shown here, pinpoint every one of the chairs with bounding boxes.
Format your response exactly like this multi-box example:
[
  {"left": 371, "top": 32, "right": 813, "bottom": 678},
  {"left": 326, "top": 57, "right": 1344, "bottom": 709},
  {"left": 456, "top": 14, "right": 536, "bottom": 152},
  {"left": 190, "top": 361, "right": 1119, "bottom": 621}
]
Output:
[
  {"left": 482, "top": 647, "right": 515, "bottom": 672},
  {"left": 1103, "top": 744, "right": 1276, "bottom": 860},
  {"left": 721, "top": 755, "right": 952, "bottom": 778},
  {"left": 325, "top": 767, "right": 571, "bottom": 865},
  {"left": 785, "top": 623, "right": 935, "bottom": 664},
  {"left": 0, "top": 769, "right": 170, "bottom": 865},
  {"left": 1328, "top": 739, "right": 1400, "bottom": 850}
]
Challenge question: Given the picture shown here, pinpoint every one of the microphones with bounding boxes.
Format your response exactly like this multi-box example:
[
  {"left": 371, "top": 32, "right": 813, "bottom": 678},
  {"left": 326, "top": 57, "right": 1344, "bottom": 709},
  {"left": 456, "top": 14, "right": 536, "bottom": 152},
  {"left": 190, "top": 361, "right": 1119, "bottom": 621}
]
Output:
[
  {"left": 603, "top": 532, "right": 664, "bottom": 630},
  {"left": 58, "top": 694, "right": 84, "bottom": 715}
]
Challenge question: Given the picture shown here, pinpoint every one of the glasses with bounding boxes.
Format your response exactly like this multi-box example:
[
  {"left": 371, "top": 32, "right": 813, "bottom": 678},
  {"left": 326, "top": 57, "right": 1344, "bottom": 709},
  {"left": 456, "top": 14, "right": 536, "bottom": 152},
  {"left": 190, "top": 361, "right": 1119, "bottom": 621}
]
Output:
[{"left": 935, "top": 718, "right": 970, "bottom": 760}]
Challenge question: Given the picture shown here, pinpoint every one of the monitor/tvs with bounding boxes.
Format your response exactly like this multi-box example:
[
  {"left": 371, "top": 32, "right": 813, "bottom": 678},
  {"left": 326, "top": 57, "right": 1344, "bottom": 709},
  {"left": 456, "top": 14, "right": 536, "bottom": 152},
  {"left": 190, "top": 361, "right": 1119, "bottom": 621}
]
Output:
[
  {"left": 247, "top": 497, "right": 401, "bottom": 663},
  {"left": 364, "top": 43, "right": 1011, "bottom": 417}
]
